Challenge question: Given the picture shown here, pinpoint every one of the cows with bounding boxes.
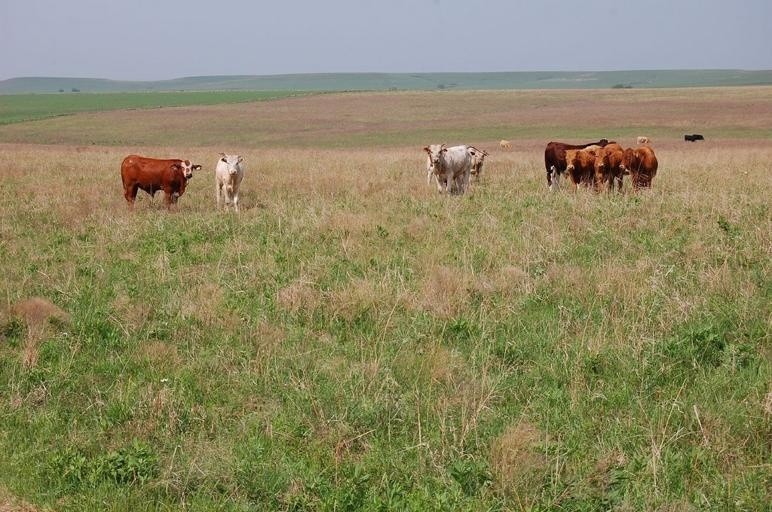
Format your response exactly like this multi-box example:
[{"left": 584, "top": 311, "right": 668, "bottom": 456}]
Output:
[
  {"left": 422, "top": 143, "right": 489, "bottom": 197},
  {"left": 121, "top": 155, "right": 202, "bottom": 211},
  {"left": 684, "top": 134, "right": 703, "bottom": 143},
  {"left": 215, "top": 152, "right": 245, "bottom": 214},
  {"left": 499, "top": 139, "right": 512, "bottom": 151},
  {"left": 544, "top": 136, "right": 657, "bottom": 195}
]
